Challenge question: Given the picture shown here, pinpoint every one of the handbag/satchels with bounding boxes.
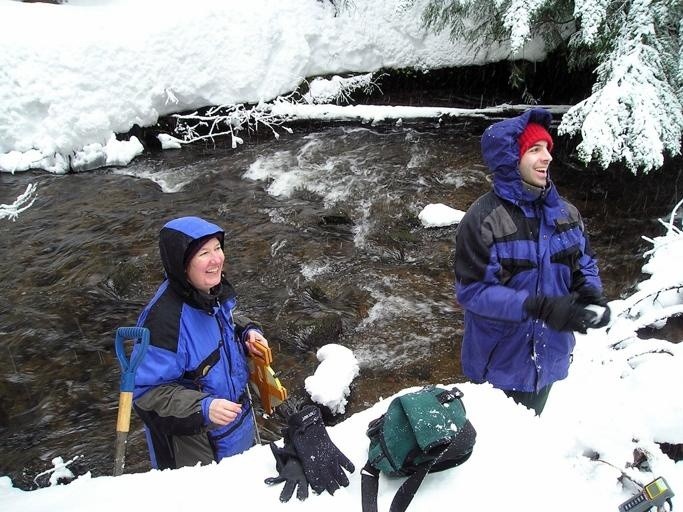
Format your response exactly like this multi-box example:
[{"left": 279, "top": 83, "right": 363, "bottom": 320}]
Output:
[{"left": 366, "top": 387, "right": 476, "bottom": 478}]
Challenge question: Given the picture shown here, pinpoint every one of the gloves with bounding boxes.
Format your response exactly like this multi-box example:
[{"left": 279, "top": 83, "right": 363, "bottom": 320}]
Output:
[
  {"left": 264, "top": 404, "right": 355, "bottom": 503},
  {"left": 525, "top": 287, "right": 611, "bottom": 335}
]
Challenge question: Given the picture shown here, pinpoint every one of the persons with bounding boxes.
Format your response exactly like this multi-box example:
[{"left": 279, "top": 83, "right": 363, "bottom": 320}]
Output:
[
  {"left": 127, "top": 216, "right": 269, "bottom": 468},
  {"left": 453, "top": 105, "right": 610, "bottom": 416}
]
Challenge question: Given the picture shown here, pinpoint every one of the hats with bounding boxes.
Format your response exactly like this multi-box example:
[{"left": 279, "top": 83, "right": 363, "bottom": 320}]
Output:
[{"left": 517, "top": 120, "right": 554, "bottom": 164}]
